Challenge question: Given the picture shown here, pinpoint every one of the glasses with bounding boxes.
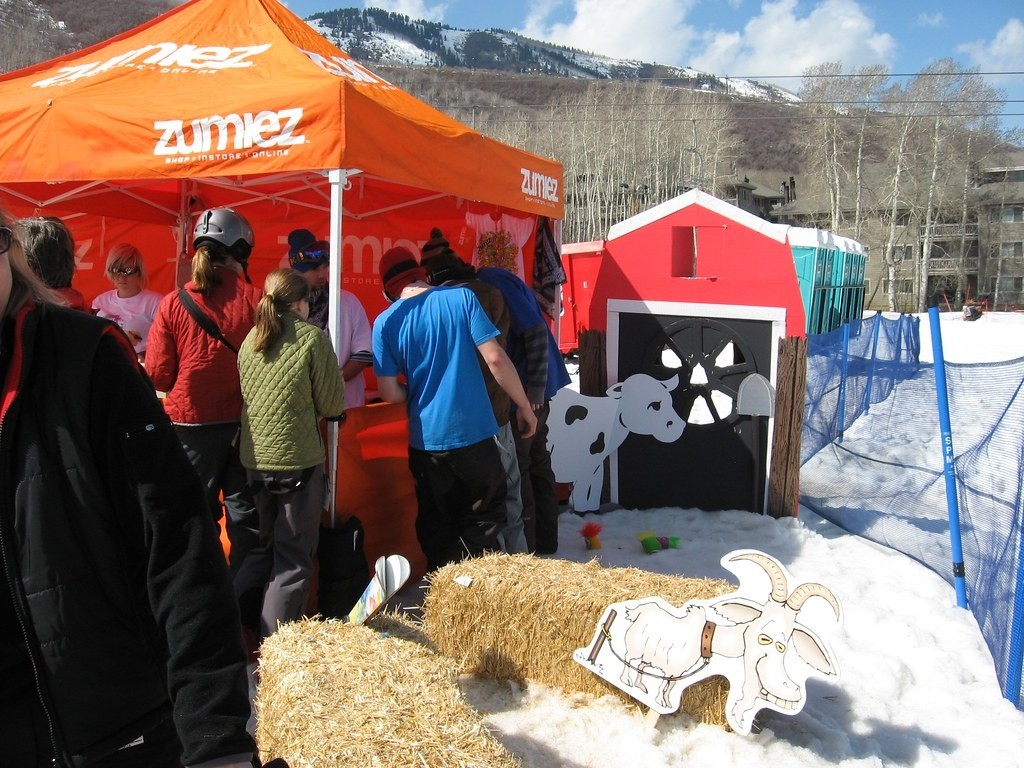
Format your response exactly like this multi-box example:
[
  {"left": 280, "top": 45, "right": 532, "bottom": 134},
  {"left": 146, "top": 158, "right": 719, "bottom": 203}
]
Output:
[
  {"left": 382, "top": 289, "right": 396, "bottom": 303},
  {"left": 0, "top": 226, "right": 13, "bottom": 255},
  {"left": 112, "top": 267, "right": 138, "bottom": 276},
  {"left": 302, "top": 241, "right": 329, "bottom": 259}
]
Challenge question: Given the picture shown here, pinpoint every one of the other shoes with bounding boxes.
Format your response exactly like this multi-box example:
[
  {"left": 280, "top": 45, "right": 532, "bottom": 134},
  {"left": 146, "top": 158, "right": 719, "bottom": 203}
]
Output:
[
  {"left": 243, "top": 623, "right": 260, "bottom": 647},
  {"left": 538, "top": 542, "right": 558, "bottom": 554}
]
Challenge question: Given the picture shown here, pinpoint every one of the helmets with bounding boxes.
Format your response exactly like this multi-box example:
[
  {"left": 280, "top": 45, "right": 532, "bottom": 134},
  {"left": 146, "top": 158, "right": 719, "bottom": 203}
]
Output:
[{"left": 193, "top": 206, "right": 255, "bottom": 264}]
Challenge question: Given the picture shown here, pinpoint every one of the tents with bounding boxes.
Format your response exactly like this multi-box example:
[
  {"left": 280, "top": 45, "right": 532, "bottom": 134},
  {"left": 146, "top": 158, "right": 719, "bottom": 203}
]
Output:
[{"left": 0, "top": 0, "right": 566, "bottom": 595}]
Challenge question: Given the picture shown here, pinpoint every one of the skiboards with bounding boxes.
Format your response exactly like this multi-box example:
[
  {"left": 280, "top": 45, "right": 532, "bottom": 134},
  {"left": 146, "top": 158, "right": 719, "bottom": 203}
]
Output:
[{"left": 346, "top": 554, "right": 412, "bottom": 625}]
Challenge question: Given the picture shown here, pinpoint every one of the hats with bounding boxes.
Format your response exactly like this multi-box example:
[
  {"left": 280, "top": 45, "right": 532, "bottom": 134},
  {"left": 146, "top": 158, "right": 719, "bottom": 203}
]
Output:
[
  {"left": 379, "top": 246, "right": 426, "bottom": 296},
  {"left": 420, "top": 228, "right": 454, "bottom": 266},
  {"left": 287, "top": 228, "right": 330, "bottom": 273}
]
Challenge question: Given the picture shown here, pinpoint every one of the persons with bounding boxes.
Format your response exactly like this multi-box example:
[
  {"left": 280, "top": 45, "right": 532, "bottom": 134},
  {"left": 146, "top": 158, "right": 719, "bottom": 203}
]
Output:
[
  {"left": 0, "top": 204, "right": 572, "bottom": 768},
  {"left": 961, "top": 298, "right": 984, "bottom": 321}
]
